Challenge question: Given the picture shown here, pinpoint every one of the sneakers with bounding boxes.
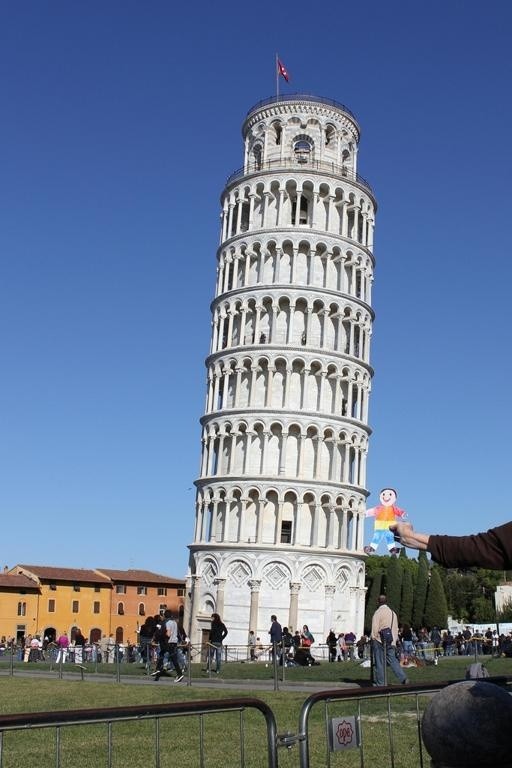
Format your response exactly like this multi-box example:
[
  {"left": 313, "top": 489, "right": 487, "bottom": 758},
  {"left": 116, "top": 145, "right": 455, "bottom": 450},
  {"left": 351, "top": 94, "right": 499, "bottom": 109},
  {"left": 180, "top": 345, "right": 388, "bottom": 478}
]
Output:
[
  {"left": 403, "top": 678, "right": 410, "bottom": 685},
  {"left": 149, "top": 670, "right": 161, "bottom": 676},
  {"left": 174, "top": 674, "right": 185, "bottom": 683}
]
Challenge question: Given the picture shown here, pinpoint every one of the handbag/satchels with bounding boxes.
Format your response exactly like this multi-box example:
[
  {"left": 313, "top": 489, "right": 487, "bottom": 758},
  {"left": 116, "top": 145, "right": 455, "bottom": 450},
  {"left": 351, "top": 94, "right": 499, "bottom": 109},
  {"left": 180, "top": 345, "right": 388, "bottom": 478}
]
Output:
[{"left": 380, "top": 611, "right": 394, "bottom": 644}]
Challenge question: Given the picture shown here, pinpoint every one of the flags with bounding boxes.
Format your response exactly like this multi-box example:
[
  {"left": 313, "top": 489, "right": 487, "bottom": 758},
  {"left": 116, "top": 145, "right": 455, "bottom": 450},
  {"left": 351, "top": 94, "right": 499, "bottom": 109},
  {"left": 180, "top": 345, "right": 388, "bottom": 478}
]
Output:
[{"left": 278, "top": 59, "right": 288, "bottom": 83}]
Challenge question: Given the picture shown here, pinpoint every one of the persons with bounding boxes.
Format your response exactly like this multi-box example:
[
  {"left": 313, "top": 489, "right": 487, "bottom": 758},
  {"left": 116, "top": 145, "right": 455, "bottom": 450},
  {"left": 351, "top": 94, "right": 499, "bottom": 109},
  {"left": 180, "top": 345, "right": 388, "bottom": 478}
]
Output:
[{"left": 389, "top": 522, "right": 512, "bottom": 570}]
